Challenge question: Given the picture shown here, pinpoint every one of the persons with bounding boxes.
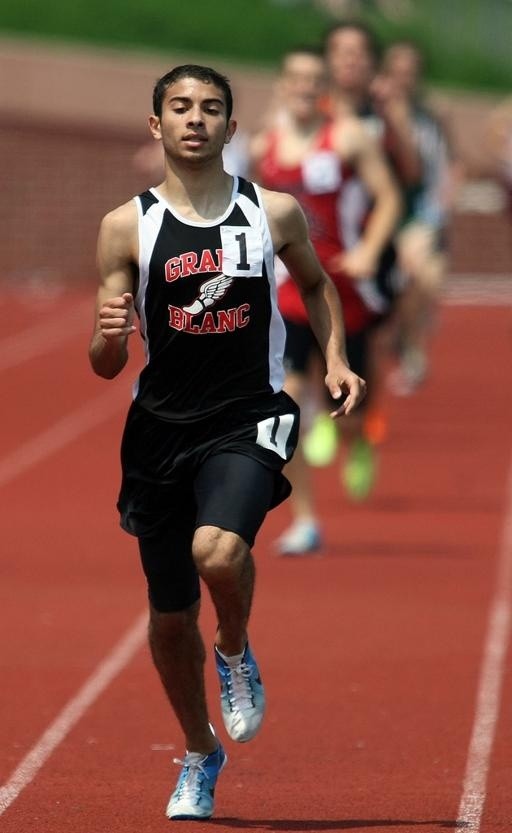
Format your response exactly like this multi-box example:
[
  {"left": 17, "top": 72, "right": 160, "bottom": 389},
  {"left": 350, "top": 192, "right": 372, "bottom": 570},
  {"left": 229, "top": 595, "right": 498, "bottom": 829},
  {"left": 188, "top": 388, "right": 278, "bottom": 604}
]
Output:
[
  {"left": 83, "top": 60, "right": 370, "bottom": 823},
  {"left": 125, "top": 16, "right": 469, "bottom": 554}
]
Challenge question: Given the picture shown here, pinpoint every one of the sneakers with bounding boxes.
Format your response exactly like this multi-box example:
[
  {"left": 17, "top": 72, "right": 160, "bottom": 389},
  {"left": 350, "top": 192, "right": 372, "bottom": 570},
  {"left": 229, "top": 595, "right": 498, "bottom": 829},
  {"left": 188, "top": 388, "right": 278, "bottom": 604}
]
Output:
[
  {"left": 214, "top": 634, "right": 266, "bottom": 744},
  {"left": 279, "top": 522, "right": 318, "bottom": 557},
  {"left": 166, "top": 722, "right": 228, "bottom": 820}
]
